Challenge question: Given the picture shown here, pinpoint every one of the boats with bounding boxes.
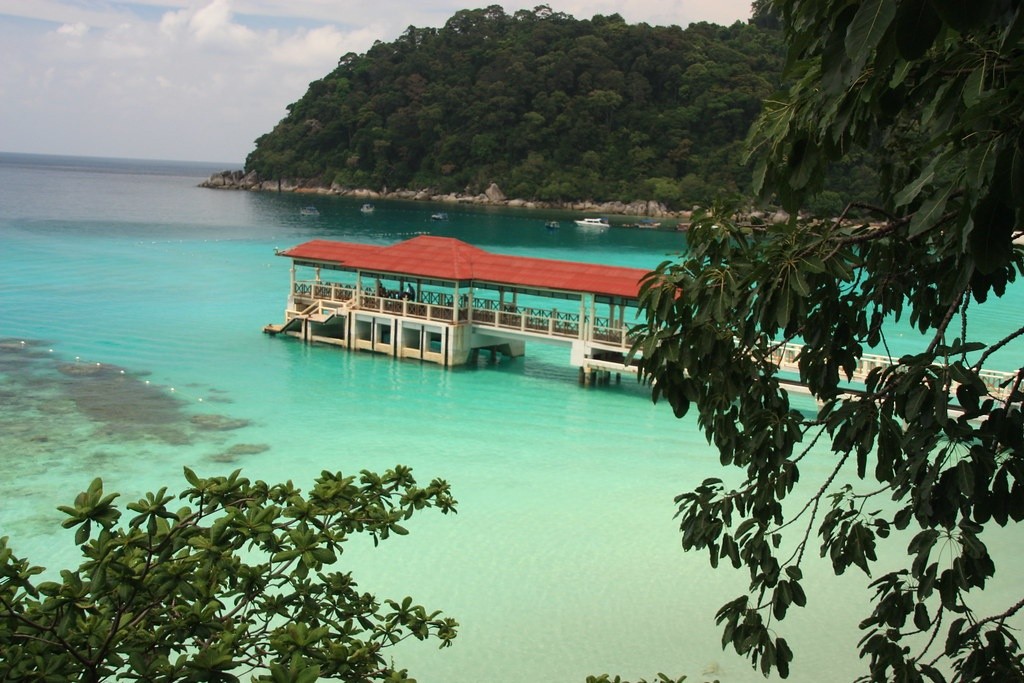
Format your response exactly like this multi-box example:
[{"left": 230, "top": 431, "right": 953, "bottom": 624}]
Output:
[
  {"left": 299, "top": 207, "right": 320, "bottom": 216},
  {"left": 546, "top": 221, "right": 559, "bottom": 229},
  {"left": 432, "top": 213, "right": 448, "bottom": 220},
  {"left": 363, "top": 204, "right": 374, "bottom": 212}
]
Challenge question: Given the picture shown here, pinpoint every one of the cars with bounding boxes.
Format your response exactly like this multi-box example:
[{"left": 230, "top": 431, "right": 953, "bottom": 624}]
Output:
[{"left": 574, "top": 216, "right": 610, "bottom": 228}]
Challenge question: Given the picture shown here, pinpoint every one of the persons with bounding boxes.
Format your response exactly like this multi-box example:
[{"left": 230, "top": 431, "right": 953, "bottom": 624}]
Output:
[
  {"left": 406, "top": 284, "right": 415, "bottom": 301},
  {"left": 377, "top": 284, "right": 385, "bottom": 297},
  {"left": 459, "top": 294, "right": 468, "bottom": 307}
]
